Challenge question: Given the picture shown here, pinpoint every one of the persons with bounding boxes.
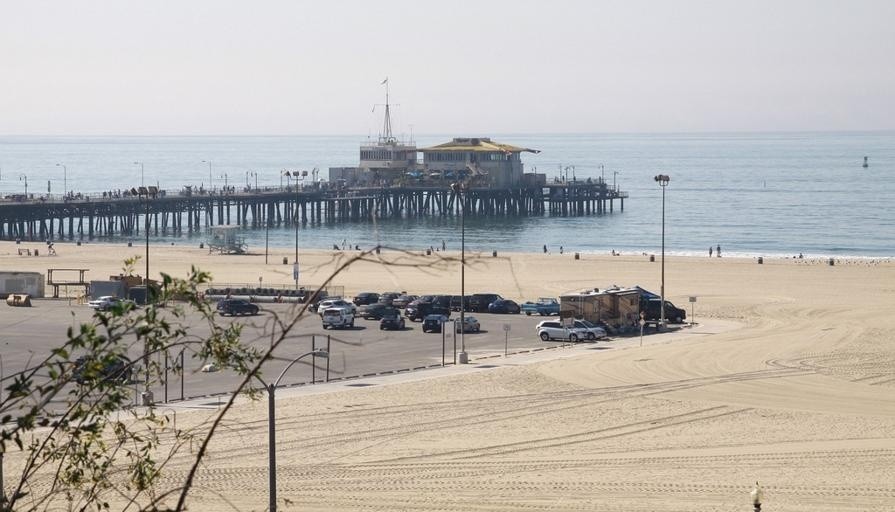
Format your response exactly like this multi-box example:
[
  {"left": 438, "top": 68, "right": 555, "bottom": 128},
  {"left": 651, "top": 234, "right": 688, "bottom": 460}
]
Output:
[
  {"left": 543, "top": 244, "right": 548, "bottom": 254},
  {"left": 717, "top": 244, "right": 721, "bottom": 257},
  {"left": 356, "top": 245, "right": 361, "bottom": 250},
  {"left": 559, "top": 245, "right": 564, "bottom": 256},
  {"left": 708, "top": 247, "right": 713, "bottom": 258},
  {"left": 333, "top": 243, "right": 340, "bottom": 250},
  {"left": 441, "top": 240, "right": 446, "bottom": 252},
  {"left": 342, "top": 239, "right": 347, "bottom": 249},
  {"left": 48, "top": 243, "right": 55, "bottom": 255}
]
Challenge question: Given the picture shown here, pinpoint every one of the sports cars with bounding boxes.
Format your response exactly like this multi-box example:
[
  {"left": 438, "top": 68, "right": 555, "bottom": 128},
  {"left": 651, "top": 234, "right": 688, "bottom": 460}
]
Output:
[{"left": 520, "top": 297, "right": 560, "bottom": 316}]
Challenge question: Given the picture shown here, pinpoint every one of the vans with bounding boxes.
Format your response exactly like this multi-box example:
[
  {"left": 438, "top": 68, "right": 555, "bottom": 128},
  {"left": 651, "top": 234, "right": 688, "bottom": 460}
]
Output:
[{"left": 534, "top": 319, "right": 590, "bottom": 343}]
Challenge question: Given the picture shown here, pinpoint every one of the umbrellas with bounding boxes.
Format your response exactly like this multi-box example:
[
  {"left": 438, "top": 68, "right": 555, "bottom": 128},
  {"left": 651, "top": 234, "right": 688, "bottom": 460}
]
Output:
[
  {"left": 442, "top": 171, "right": 453, "bottom": 177},
  {"left": 429, "top": 172, "right": 440, "bottom": 176},
  {"left": 405, "top": 170, "right": 424, "bottom": 178}
]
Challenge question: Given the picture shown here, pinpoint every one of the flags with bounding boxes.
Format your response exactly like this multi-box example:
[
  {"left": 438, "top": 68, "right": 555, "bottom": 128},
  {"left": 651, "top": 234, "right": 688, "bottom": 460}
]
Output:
[{"left": 381, "top": 79, "right": 387, "bottom": 86}]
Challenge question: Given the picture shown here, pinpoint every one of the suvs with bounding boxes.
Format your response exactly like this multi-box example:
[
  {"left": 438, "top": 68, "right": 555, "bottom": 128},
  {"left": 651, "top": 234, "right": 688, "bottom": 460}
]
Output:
[
  {"left": 216, "top": 299, "right": 260, "bottom": 316},
  {"left": 646, "top": 298, "right": 688, "bottom": 324},
  {"left": 469, "top": 294, "right": 504, "bottom": 312}
]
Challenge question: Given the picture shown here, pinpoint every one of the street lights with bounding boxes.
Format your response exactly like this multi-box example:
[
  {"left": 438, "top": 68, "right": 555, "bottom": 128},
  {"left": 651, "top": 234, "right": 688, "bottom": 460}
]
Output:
[
  {"left": 531, "top": 160, "right": 620, "bottom": 195},
  {"left": 651, "top": 172, "right": 674, "bottom": 337},
  {"left": 129, "top": 185, "right": 161, "bottom": 406},
  {"left": 18, "top": 172, "right": 28, "bottom": 200},
  {"left": 446, "top": 177, "right": 472, "bottom": 366},
  {"left": 751, "top": 480, "right": 763, "bottom": 512},
  {"left": 199, "top": 158, "right": 322, "bottom": 193},
  {"left": 133, "top": 160, "right": 145, "bottom": 186},
  {"left": 55, "top": 162, "right": 66, "bottom": 195},
  {"left": 198, "top": 347, "right": 335, "bottom": 512},
  {"left": 282, "top": 168, "right": 311, "bottom": 290}
]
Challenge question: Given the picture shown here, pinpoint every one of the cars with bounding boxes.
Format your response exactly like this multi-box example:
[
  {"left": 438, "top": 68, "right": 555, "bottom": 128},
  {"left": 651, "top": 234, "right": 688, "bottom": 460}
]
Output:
[
  {"left": 307, "top": 297, "right": 355, "bottom": 330},
  {"left": 88, "top": 295, "right": 136, "bottom": 311},
  {"left": 379, "top": 313, "right": 406, "bottom": 330},
  {"left": 377, "top": 292, "right": 468, "bottom": 321},
  {"left": 359, "top": 303, "right": 401, "bottom": 320},
  {"left": 422, "top": 314, "right": 445, "bottom": 332},
  {"left": 454, "top": 316, "right": 481, "bottom": 333},
  {"left": 352, "top": 292, "right": 381, "bottom": 307},
  {"left": 553, "top": 318, "right": 608, "bottom": 341},
  {"left": 488, "top": 299, "right": 521, "bottom": 314}
]
